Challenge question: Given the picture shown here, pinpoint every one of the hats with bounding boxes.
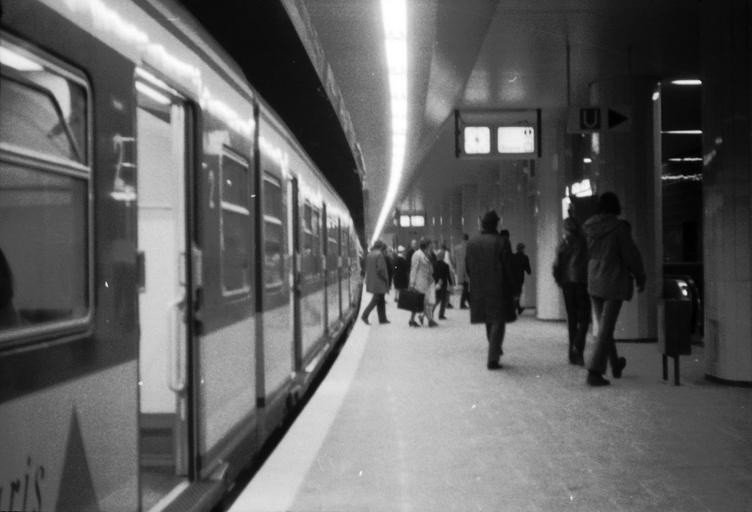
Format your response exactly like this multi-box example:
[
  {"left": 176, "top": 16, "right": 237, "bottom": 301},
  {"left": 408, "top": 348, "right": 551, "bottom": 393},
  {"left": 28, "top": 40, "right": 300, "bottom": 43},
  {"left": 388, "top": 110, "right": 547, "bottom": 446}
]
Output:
[{"left": 482, "top": 211, "right": 500, "bottom": 229}]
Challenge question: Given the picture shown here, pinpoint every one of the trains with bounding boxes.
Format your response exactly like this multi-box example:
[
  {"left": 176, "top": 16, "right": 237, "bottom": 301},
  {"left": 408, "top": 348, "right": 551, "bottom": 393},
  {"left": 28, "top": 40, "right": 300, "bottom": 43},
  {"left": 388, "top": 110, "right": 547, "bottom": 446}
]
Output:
[{"left": 0, "top": 1, "right": 365, "bottom": 511}]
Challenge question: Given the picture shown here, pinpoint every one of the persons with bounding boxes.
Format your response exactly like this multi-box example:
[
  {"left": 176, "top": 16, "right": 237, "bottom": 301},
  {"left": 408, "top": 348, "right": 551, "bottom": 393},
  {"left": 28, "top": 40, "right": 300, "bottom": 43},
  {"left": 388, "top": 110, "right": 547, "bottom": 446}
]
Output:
[
  {"left": 362, "top": 229, "right": 533, "bottom": 327},
  {"left": 579, "top": 192, "right": 648, "bottom": 387},
  {"left": 465, "top": 211, "right": 513, "bottom": 370},
  {"left": 552, "top": 216, "right": 588, "bottom": 365}
]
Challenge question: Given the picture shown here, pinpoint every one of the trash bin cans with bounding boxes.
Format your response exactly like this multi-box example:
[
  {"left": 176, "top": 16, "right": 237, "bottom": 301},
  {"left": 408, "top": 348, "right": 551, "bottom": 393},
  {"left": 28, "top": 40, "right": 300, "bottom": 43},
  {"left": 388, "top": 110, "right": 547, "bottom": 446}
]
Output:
[{"left": 657, "top": 278, "right": 693, "bottom": 386}]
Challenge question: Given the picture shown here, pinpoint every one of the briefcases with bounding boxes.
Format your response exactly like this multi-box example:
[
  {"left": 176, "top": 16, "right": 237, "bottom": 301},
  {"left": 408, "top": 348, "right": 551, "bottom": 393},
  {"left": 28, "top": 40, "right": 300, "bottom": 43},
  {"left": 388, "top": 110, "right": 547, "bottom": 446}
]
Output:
[{"left": 398, "top": 290, "right": 425, "bottom": 313}]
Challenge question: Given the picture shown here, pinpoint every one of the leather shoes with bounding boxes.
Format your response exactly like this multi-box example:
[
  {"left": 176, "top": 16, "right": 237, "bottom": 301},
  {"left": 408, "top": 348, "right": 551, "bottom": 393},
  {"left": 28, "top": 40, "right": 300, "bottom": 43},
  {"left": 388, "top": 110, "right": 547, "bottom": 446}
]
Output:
[
  {"left": 613, "top": 357, "right": 627, "bottom": 378},
  {"left": 587, "top": 372, "right": 610, "bottom": 387}
]
